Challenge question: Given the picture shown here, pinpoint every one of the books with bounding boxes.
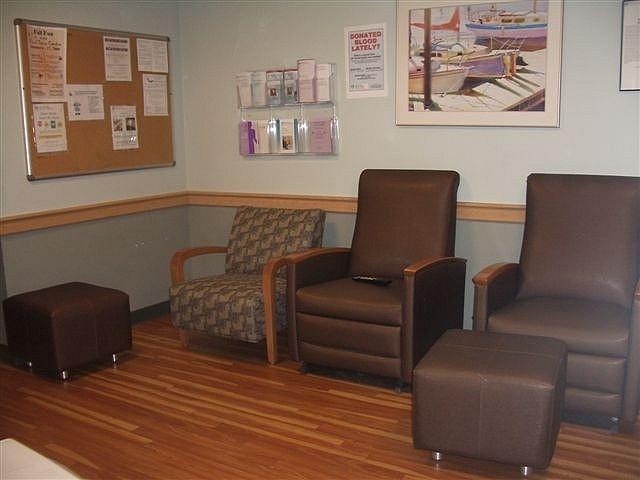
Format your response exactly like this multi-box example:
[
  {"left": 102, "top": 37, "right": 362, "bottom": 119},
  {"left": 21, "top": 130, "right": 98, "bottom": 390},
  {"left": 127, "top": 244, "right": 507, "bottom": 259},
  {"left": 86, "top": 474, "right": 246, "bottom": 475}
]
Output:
[{"left": 232, "top": 58, "right": 333, "bottom": 156}]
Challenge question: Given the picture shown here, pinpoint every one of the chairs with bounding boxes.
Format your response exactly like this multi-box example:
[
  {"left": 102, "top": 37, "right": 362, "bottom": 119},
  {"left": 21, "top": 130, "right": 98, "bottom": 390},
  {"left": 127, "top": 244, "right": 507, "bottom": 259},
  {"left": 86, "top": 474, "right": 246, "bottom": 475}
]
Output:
[
  {"left": 473, "top": 172, "right": 640, "bottom": 429},
  {"left": 281, "top": 168, "right": 468, "bottom": 390},
  {"left": 167, "top": 202, "right": 328, "bottom": 370}
]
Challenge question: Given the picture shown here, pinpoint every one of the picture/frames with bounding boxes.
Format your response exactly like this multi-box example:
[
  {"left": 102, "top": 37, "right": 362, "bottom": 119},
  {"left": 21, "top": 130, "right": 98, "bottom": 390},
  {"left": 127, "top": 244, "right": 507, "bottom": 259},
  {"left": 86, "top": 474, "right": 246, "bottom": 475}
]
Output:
[{"left": 396, "top": 1, "right": 564, "bottom": 128}]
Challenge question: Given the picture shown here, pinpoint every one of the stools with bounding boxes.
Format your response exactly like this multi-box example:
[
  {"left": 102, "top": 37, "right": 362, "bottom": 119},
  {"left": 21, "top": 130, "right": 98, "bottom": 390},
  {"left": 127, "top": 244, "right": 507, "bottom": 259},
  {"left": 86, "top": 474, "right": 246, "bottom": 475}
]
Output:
[
  {"left": 3, "top": 275, "right": 140, "bottom": 380},
  {"left": 410, "top": 328, "right": 571, "bottom": 478}
]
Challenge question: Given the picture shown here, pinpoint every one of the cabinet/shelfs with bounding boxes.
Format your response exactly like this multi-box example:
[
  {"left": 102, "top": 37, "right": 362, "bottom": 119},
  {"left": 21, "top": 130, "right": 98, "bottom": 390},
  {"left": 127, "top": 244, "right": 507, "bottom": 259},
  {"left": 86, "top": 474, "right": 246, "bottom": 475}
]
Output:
[{"left": 237, "top": 99, "right": 337, "bottom": 158}]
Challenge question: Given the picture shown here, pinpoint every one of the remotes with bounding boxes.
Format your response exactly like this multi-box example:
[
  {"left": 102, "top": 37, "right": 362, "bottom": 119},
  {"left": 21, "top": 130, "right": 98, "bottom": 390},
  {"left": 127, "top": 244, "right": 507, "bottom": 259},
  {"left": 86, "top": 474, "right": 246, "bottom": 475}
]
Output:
[{"left": 352, "top": 275, "right": 392, "bottom": 286}]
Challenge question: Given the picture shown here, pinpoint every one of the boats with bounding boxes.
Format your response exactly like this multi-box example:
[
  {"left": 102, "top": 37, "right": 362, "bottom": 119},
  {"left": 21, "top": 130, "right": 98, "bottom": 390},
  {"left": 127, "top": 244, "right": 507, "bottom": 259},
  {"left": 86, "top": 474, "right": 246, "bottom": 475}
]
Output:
[
  {"left": 482, "top": 38, "right": 546, "bottom": 50},
  {"left": 421, "top": 40, "right": 520, "bottom": 77},
  {"left": 409, "top": 36, "right": 470, "bottom": 93},
  {"left": 465, "top": 4, "right": 547, "bottom": 38}
]
